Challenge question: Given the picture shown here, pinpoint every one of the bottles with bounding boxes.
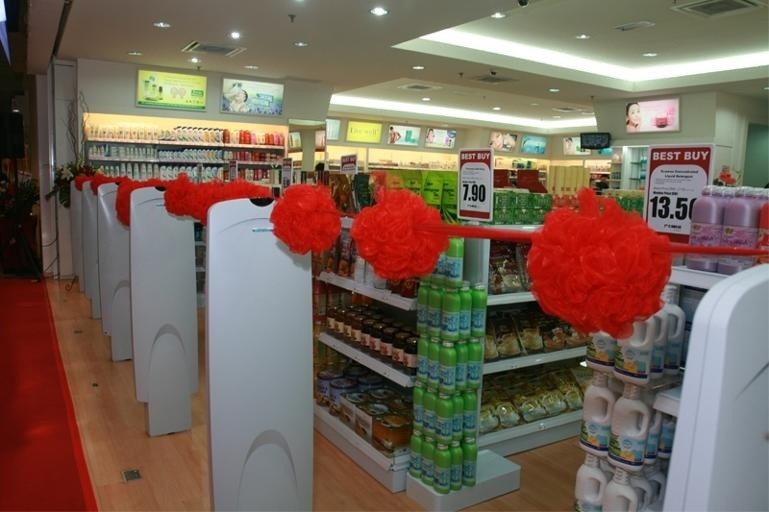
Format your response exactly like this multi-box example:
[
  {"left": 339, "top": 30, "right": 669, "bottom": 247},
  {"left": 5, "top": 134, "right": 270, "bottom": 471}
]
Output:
[
  {"left": 408, "top": 235, "right": 488, "bottom": 494},
  {"left": 686, "top": 185, "right": 769, "bottom": 278}
]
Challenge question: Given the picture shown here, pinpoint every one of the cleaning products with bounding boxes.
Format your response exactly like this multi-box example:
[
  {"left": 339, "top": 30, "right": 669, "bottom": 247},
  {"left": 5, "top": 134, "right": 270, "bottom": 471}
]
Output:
[{"left": 571, "top": 185, "right": 769, "bottom": 511}]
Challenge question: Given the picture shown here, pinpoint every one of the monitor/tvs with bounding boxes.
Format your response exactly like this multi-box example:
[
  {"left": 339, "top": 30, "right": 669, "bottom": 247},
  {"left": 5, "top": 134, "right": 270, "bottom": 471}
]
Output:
[{"left": 579, "top": 132, "right": 610, "bottom": 149}]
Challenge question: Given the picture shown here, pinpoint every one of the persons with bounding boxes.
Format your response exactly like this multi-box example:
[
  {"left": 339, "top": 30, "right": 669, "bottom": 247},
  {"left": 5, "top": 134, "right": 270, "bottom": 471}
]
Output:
[
  {"left": 489, "top": 130, "right": 515, "bottom": 151},
  {"left": 563, "top": 137, "right": 574, "bottom": 154},
  {"left": 625, "top": 102, "right": 640, "bottom": 133},
  {"left": 426, "top": 129, "right": 436, "bottom": 145},
  {"left": 389, "top": 127, "right": 401, "bottom": 144}
]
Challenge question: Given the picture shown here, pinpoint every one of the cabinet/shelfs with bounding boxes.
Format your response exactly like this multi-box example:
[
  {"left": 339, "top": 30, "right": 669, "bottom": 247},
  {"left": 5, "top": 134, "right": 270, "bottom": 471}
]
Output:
[{"left": 85, "top": 137, "right": 769, "bottom": 512}]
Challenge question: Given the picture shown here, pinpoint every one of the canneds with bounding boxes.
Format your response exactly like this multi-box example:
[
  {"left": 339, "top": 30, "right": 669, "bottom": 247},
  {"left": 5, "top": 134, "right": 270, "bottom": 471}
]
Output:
[{"left": 317, "top": 302, "right": 419, "bottom": 451}]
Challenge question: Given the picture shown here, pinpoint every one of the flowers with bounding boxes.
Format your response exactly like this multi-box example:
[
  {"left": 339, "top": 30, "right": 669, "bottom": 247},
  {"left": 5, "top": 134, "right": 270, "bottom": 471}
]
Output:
[
  {"left": 45, "top": 153, "right": 104, "bottom": 207},
  {"left": 1, "top": 178, "right": 40, "bottom": 219}
]
